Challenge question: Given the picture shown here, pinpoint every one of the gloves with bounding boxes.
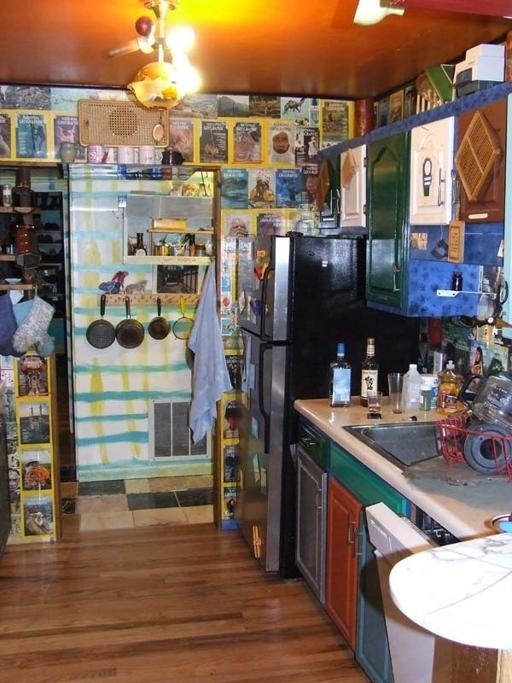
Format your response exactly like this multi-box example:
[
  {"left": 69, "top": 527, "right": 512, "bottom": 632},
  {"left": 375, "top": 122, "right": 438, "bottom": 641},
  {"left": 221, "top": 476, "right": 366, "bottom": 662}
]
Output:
[{"left": 0, "top": 295, "right": 55, "bottom": 360}]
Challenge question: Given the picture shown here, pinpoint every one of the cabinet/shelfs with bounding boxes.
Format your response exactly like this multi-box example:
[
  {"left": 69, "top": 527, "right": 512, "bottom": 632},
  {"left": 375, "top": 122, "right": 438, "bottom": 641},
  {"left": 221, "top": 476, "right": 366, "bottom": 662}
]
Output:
[
  {"left": 365, "top": 131, "right": 484, "bottom": 318},
  {"left": 407, "top": 95, "right": 506, "bottom": 227},
  {"left": 290, "top": 422, "right": 413, "bottom": 683},
  {"left": 313, "top": 144, "right": 367, "bottom": 236}
]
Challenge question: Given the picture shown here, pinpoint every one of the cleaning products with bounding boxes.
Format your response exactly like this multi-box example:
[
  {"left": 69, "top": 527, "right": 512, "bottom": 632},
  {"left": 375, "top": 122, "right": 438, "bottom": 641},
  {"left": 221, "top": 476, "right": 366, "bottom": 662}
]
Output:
[{"left": 402, "top": 362, "right": 420, "bottom": 412}]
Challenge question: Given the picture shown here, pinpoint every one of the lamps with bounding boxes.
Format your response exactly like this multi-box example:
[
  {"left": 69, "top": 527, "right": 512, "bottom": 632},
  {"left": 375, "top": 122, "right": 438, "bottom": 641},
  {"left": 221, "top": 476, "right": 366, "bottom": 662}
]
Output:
[{"left": 125, "top": 0, "right": 188, "bottom": 112}]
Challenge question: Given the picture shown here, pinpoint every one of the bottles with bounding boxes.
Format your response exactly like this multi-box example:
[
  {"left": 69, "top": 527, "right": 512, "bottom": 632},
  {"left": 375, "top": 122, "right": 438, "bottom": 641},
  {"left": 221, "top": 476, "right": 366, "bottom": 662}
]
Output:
[
  {"left": 328, "top": 343, "right": 352, "bottom": 408},
  {"left": 2, "top": 184, "right": 13, "bottom": 207},
  {"left": 60, "top": 142, "right": 77, "bottom": 163},
  {"left": 402, "top": 364, "right": 424, "bottom": 412},
  {"left": 435, "top": 358, "right": 464, "bottom": 415},
  {"left": 360, "top": 338, "right": 380, "bottom": 407},
  {"left": 133, "top": 233, "right": 147, "bottom": 257}
]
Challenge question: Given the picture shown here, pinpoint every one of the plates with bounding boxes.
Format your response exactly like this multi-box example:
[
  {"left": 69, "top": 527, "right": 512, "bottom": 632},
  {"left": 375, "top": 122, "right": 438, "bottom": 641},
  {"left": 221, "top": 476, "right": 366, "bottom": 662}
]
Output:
[{"left": 4, "top": 278, "right": 22, "bottom": 285}]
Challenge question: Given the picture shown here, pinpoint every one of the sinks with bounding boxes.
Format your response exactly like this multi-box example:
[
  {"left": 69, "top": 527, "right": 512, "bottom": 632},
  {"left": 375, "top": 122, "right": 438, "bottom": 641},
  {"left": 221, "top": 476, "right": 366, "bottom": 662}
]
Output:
[{"left": 342, "top": 418, "right": 440, "bottom": 470}]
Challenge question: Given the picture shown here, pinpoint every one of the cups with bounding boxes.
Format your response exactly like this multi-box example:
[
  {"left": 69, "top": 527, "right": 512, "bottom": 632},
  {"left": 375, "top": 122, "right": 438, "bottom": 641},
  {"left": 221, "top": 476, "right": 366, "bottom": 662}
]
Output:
[
  {"left": 366, "top": 391, "right": 383, "bottom": 413},
  {"left": 86, "top": 144, "right": 104, "bottom": 164},
  {"left": 117, "top": 146, "right": 135, "bottom": 165},
  {"left": 138, "top": 146, "right": 156, "bottom": 164},
  {"left": 386, "top": 372, "right": 404, "bottom": 415}
]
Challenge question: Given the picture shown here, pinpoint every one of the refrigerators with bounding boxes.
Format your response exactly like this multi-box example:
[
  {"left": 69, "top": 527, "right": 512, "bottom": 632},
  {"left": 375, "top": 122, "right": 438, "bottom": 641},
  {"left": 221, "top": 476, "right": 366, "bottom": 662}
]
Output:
[{"left": 234, "top": 227, "right": 422, "bottom": 577}]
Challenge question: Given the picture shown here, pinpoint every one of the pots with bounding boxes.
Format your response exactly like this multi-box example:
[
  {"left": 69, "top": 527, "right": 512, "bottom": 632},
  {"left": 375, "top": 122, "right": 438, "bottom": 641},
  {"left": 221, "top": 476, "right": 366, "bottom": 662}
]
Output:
[
  {"left": 148, "top": 296, "right": 171, "bottom": 340},
  {"left": 86, "top": 293, "right": 116, "bottom": 349},
  {"left": 114, "top": 296, "right": 145, "bottom": 349}
]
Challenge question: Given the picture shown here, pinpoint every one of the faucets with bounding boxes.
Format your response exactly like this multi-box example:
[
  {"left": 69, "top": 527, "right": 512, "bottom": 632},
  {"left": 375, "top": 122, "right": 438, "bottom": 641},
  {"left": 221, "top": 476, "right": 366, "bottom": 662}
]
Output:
[{"left": 458, "top": 372, "right": 485, "bottom": 403}]
